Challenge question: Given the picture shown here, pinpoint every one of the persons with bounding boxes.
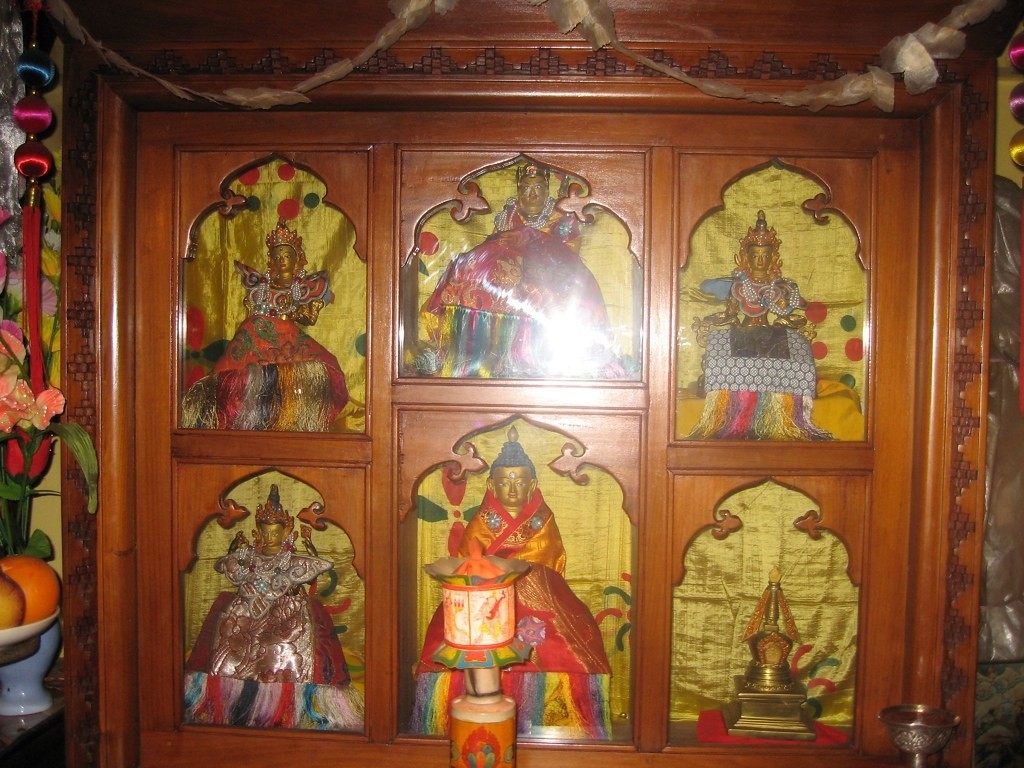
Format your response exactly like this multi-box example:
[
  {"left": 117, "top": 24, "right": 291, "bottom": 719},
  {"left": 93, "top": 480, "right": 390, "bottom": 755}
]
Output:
[
  {"left": 422, "top": 160, "right": 614, "bottom": 377},
  {"left": 184, "top": 485, "right": 366, "bottom": 730},
  {"left": 181, "top": 222, "right": 351, "bottom": 435},
  {"left": 686, "top": 222, "right": 832, "bottom": 442},
  {"left": 412, "top": 441, "right": 613, "bottom": 742}
]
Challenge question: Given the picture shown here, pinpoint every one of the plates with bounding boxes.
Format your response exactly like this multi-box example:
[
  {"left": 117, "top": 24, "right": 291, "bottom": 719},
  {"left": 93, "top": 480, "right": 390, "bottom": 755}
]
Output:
[
  {"left": 424, "top": 556, "right": 532, "bottom": 585},
  {"left": 431, "top": 643, "right": 532, "bottom": 668},
  {"left": 0, "top": 605, "right": 60, "bottom": 648}
]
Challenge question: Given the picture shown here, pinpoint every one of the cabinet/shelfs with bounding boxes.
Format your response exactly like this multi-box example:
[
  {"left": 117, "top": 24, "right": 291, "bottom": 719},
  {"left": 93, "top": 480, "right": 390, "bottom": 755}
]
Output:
[{"left": 94, "top": 0, "right": 1024, "bottom": 768}]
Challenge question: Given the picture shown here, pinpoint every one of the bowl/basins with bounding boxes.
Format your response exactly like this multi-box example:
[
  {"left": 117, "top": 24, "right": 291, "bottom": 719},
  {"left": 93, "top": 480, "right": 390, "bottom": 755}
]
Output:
[{"left": 442, "top": 584, "right": 516, "bottom": 649}]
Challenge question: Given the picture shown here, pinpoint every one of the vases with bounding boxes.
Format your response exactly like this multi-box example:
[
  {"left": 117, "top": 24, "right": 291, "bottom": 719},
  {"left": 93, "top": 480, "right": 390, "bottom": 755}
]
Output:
[{"left": 0, "top": 618, "right": 61, "bottom": 716}]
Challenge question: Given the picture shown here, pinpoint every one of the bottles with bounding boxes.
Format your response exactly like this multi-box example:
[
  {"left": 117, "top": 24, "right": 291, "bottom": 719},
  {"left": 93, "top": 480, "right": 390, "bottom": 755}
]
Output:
[{"left": 450, "top": 694, "right": 516, "bottom": 768}]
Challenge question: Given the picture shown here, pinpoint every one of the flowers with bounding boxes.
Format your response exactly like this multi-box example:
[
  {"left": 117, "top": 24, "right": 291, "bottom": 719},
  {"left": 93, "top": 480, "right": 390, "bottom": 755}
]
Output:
[{"left": 0, "top": 211, "right": 98, "bottom": 560}]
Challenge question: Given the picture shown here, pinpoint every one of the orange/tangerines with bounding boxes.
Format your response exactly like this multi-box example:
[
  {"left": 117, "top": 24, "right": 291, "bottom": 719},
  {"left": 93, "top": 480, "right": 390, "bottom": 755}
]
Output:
[{"left": 0, "top": 556, "right": 60, "bottom": 628}]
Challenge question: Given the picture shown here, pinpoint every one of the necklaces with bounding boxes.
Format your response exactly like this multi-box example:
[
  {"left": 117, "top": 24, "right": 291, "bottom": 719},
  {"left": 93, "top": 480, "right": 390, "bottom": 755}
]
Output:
[
  {"left": 500, "top": 197, "right": 556, "bottom": 229},
  {"left": 255, "top": 269, "right": 306, "bottom": 317},
  {"left": 743, "top": 277, "right": 801, "bottom": 315},
  {"left": 238, "top": 533, "right": 294, "bottom": 576}
]
